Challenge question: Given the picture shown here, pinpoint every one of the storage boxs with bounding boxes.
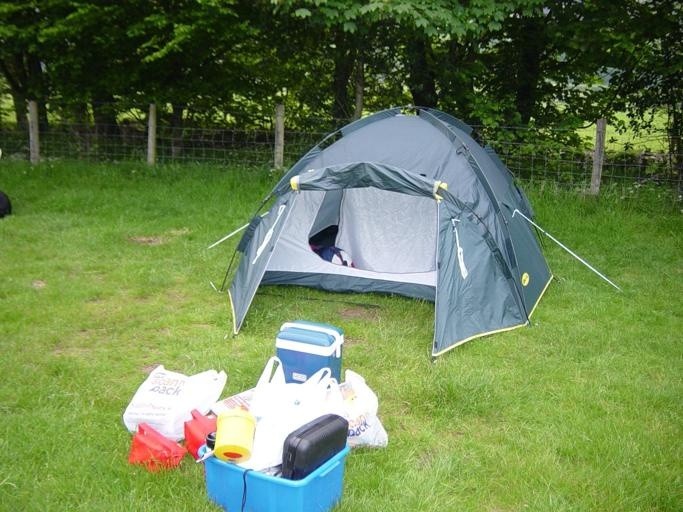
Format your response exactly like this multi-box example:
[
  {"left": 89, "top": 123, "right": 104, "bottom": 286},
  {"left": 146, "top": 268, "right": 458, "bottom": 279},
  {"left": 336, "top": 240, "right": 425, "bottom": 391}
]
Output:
[{"left": 204, "top": 443, "right": 350, "bottom": 510}]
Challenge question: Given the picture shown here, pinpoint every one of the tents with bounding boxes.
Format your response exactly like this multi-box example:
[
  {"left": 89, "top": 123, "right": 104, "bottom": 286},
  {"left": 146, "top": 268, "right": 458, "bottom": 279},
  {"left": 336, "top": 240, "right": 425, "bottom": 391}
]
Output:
[{"left": 217, "top": 102, "right": 554, "bottom": 361}]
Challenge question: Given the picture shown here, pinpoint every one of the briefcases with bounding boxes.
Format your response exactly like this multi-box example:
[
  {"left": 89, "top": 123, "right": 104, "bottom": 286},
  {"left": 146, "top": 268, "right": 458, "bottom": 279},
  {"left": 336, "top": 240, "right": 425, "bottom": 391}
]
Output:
[{"left": 283, "top": 415, "right": 347, "bottom": 480}]
